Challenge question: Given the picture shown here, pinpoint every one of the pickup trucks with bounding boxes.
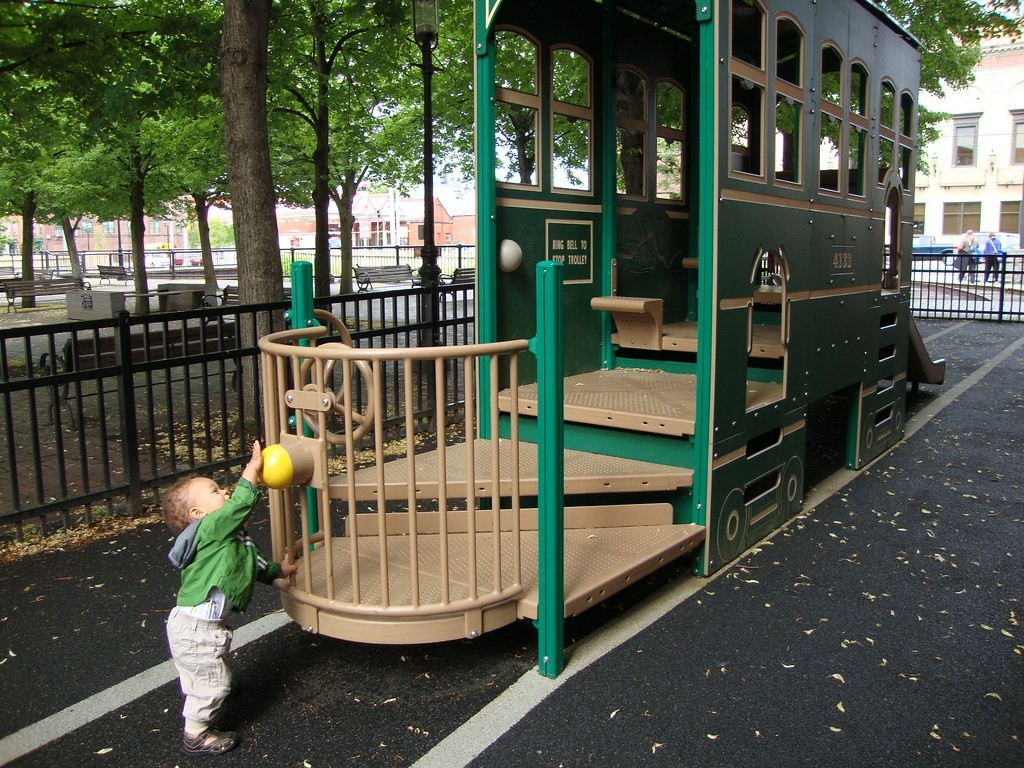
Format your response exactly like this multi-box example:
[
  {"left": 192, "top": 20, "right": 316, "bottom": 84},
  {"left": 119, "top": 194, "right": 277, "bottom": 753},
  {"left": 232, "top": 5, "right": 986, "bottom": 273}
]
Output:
[{"left": 885, "top": 235, "right": 960, "bottom": 266}]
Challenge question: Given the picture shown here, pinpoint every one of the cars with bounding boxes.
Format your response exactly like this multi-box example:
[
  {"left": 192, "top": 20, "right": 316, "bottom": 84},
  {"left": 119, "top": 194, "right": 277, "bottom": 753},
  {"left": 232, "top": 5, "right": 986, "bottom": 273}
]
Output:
[
  {"left": 961, "top": 230, "right": 1020, "bottom": 264},
  {"left": 192, "top": 257, "right": 203, "bottom": 267},
  {"left": 157, "top": 243, "right": 177, "bottom": 249}
]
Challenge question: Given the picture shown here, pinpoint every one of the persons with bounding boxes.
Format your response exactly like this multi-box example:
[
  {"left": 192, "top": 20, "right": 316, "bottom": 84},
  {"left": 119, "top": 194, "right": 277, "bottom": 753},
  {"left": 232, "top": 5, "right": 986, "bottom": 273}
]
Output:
[
  {"left": 158, "top": 439, "right": 298, "bottom": 757},
  {"left": 957, "top": 229, "right": 979, "bottom": 284},
  {"left": 982, "top": 232, "right": 1002, "bottom": 285}
]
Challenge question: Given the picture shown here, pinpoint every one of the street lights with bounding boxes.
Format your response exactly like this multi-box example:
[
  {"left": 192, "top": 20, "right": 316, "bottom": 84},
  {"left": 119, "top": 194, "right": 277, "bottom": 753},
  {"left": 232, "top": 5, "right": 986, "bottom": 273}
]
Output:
[
  {"left": 410, "top": 0, "right": 440, "bottom": 416},
  {"left": 7, "top": 241, "right": 17, "bottom": 273}
]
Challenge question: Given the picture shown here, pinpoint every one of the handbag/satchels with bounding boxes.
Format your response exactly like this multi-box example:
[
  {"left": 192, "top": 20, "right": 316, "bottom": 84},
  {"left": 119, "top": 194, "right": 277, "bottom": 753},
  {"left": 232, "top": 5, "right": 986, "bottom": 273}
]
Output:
[
  {"left": 995, "top": 251, "right": 1007, "bottom": 263},
  {"left": 969, "top": 246, "right": 982, "bottom": 261}
]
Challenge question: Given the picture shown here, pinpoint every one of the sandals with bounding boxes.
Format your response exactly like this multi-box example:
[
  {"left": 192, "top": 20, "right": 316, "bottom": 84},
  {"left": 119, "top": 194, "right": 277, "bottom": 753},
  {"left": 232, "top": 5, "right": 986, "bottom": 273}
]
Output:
[{"left": 182, "top": 728, "right": 237, "bottom": 756}]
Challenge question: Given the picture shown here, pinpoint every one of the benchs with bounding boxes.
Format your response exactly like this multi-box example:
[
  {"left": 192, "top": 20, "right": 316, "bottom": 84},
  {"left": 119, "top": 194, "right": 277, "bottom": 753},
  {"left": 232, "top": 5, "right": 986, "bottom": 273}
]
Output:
[
  {"left": 34, "top": 268, "right": 55, "bottom": 280},
  {"left": 0, "top": 278, "right": 22, "bottom": 293},
  {"left": 198, "top": 284, "right": 292, "bottom": 325},
  {"left": 0, "top": 266, "right": 19, "bottom": 278},
  {"left": 439, "top": 267, "right": 475, "bottom": 301},
  {"left": 3, "top": 277, "right": 92, "bottom": 313},
  {"left": 38, "top": 323, "right": 237, "bottom": 428},
  {"left": 97, "top": 264, "right": 134, "bottom": 285},
  {"left": 352, "top": 263, "right": 422, "bottom": 293}
]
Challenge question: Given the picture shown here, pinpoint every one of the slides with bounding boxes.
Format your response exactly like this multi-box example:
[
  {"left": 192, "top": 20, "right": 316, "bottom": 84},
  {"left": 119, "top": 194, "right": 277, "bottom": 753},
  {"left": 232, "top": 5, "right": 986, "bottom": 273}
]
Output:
[{"left": 883, "top": 280, "right": 947, "bottom": 385}]
